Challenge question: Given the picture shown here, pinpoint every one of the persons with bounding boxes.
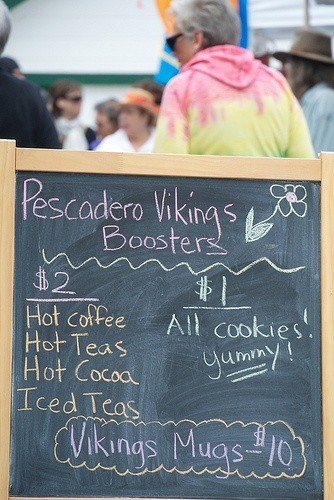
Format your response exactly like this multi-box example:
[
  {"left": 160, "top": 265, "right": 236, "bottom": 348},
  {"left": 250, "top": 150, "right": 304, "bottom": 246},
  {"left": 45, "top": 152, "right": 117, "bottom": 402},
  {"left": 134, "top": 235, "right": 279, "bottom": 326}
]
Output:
[
  {"left": 0, "top": 57, "right": 26, "bottom": 77},
  {"left": 91, "top": 83, "right": 162, "bottom": 153},
  {"left": 271, "top": 30, "right": 334, "bottom": 153},
  {"left": 51, "top": 81, "right": 89, "bottom": 151},
  {"left": 0, "top": 0, "right": 61, "bottom": 149},
  {"left": 92, "top": 98, "right": 121, "bottom": 150},
  {"left": 149, "top": 0, "right": 317, "bottom": 160}
]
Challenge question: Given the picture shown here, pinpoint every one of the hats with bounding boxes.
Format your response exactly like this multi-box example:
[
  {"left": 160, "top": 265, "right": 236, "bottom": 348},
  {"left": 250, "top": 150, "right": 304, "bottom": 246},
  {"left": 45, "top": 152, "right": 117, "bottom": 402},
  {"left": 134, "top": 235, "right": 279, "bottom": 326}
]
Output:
[
  {"left": 248, "top": 17, "right": 280, "bottom": 57},
  {"left": 272, "top": 29, "right": 334, "bottom": 62},
  {"left": 110, "top": 88, "right": 159, "bottom": 128}
]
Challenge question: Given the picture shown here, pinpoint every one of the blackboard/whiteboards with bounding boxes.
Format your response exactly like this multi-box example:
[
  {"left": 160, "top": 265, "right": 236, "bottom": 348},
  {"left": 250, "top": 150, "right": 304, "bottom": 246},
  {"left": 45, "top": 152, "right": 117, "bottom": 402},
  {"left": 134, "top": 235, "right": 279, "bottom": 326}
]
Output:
[{"left": 0, "top": 140, "right": 334, "bottom": 500}]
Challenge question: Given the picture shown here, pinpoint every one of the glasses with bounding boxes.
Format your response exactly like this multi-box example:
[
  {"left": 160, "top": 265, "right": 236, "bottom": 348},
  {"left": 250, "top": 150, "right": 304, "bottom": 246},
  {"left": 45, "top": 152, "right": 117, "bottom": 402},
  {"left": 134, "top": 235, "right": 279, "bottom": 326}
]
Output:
[
  {"left": 165, "top": 33, "right": 184, "bottom": 52},
  {"left": 97, "top": 121, "right": 111, "bottom": 127},
  {"left": 62, "top": 95, "right": 82, "bottom": 102}
]
